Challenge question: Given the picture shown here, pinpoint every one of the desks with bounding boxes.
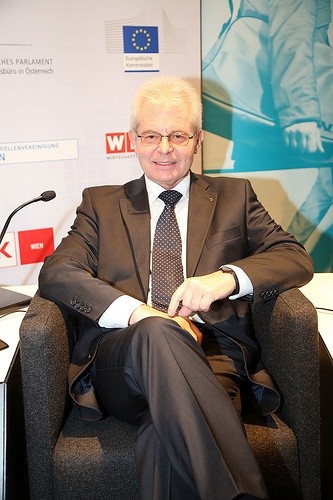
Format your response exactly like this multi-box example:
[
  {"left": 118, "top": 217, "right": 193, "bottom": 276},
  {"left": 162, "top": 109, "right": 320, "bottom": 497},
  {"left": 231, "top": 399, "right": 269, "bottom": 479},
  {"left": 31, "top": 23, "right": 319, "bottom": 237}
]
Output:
[{"left": 0, "top": 285, "right": 39, "bottom": 500}]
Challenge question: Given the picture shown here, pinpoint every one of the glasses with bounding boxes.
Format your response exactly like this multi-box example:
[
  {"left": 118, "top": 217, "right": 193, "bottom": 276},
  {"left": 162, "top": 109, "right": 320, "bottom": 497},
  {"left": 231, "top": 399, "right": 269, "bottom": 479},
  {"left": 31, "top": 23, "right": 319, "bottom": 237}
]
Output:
[{"left": 136, "top": 128, "right": 197, "bottom": 145}]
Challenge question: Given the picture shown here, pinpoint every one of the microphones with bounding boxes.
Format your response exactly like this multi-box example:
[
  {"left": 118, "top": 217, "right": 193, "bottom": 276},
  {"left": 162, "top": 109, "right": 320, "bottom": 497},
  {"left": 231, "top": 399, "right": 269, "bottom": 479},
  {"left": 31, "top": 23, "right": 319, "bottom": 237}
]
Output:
[{"left": 0, "top": 191, "right": 56, "bottom": 244}]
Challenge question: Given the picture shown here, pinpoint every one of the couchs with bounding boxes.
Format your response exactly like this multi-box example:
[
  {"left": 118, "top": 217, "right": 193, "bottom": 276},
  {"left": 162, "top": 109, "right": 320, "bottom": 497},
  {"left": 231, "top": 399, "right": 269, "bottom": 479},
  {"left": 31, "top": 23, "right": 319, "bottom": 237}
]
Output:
[{"left": 19, "top": 288, "right": 333, "bottom": 500}]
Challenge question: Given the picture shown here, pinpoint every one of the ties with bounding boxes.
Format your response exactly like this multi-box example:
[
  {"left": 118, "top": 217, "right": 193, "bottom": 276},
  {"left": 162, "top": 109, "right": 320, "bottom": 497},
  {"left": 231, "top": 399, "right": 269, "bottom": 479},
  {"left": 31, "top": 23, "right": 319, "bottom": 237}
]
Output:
[{"left": 150, "top": 189, "right": 189, "bottom": 316}]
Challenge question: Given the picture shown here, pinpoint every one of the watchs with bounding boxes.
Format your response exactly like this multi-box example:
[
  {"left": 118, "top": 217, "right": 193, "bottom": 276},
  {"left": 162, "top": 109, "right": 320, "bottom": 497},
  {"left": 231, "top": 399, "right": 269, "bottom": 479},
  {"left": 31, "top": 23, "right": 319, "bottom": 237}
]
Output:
[{"left": 220, "top": 267, "right": 239, "bottom": 293}]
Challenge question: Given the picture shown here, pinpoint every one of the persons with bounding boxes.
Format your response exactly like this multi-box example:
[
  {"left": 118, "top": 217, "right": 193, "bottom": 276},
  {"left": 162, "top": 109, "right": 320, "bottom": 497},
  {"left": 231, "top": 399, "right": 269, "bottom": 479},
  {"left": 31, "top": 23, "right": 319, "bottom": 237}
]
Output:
[
  {"left": 38, "top": 76, "right": 313, "bottom": 500},
  {"left": 232, "top": 1, "right": 332, "bottom": 273}
]
